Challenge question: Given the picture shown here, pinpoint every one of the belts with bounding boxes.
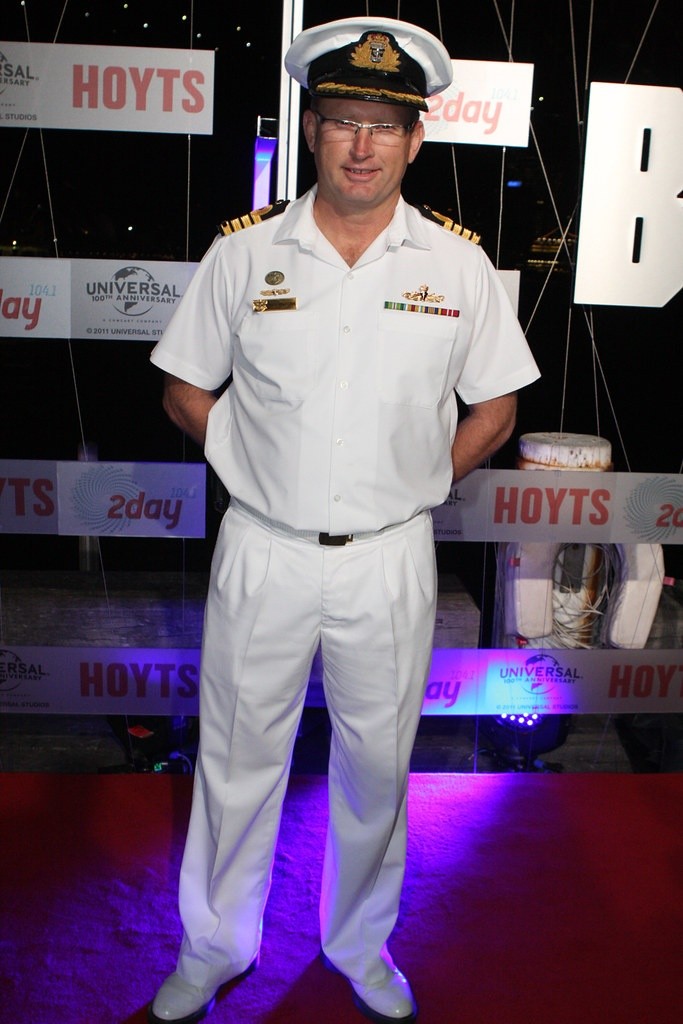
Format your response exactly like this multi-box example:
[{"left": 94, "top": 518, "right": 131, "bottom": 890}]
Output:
[{"left": 233, "top": 496, "right": 421, "bottom": 547}]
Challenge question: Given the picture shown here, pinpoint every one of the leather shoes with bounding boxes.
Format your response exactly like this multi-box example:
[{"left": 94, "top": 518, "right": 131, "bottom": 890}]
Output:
[
  {"left": 321, "top": 947, "right": 415, "bottom": 1022},
  {"left": 151, "top": 954, "right": 259, "bottom": 1023}
]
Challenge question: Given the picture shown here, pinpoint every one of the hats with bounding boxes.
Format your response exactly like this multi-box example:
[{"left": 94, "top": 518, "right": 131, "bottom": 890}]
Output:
[{"left": 284, "top": 17, "right": 453, "bottom": 113}]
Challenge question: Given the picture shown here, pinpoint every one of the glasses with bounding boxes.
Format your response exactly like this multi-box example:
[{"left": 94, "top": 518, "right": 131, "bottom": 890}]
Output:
[{"left": 311, "top": 108, "right": 417, "bottom": 145}]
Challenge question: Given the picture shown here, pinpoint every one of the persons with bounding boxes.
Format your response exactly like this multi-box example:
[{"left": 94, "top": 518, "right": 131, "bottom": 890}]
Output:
[{"left": 149, "top": 14, "right": 541, "bottom": 1024}]
